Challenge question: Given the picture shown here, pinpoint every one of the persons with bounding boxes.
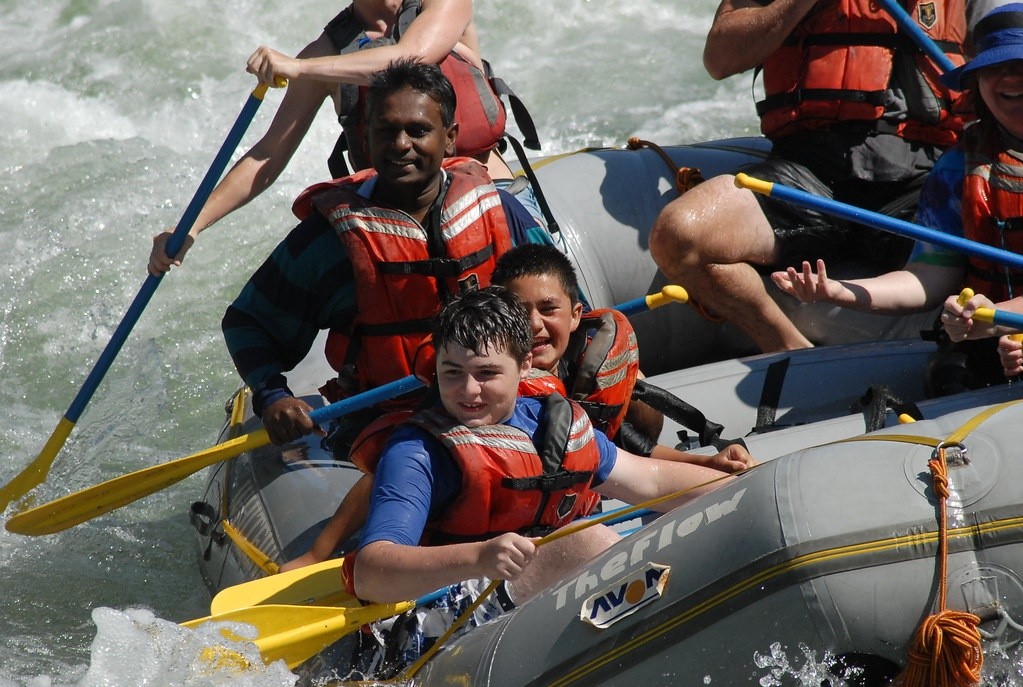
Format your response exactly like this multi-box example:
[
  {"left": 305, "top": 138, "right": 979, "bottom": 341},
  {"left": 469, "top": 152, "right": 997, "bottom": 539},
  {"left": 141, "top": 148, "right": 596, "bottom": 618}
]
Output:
[
  {"left": 221, "top": 57, "right": 590, "bottom": 445},
  {"left": 280, "top": 245, "right": 751, "bottom": 577},
  {"left": 941, "top": 294, "right": 1023, "bottom": 376},
  {"left": 650, "top": 0, "right": 1003, "bottom": 350},
  {"left": 771, "top": 4, "right": 1023, "bottom": 310},
  {"left": 148, "top": 0, "right": 548, "bottom": 275}
]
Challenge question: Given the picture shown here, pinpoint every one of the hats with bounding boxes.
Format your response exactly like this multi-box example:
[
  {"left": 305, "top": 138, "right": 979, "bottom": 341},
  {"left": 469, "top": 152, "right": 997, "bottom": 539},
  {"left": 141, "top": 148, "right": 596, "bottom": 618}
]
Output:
[{"left": 936, "top": 3, "right": 1023, "bottom": 92}]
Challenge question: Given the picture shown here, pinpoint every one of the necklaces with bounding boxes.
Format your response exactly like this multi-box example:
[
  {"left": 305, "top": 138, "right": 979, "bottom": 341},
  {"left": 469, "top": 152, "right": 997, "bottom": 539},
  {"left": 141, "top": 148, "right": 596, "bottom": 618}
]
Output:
[{"left": 354, "top": 286, "right": 737, "bottom": 651}]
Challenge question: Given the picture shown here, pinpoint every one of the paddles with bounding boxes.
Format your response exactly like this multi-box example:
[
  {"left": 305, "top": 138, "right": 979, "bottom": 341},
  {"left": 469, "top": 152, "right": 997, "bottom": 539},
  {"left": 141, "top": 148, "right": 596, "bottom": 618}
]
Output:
[
  {"left": 1, "top": 73, "right": 287, "bottom": 512},
  {"left": 174, "top": 414, "right": 919, "bottom": 671},
  {"left": 733, "top": 171, "right": 1023, "bottom": 263},
  {"left": 958, "top": 287, "right": 1022, "bottom": 329},
  {"left": 878, "top": 0, "right": 957, "bottom": 72},
  {"left": 4, "top": 282, "right": 689, "bottom": 537}
]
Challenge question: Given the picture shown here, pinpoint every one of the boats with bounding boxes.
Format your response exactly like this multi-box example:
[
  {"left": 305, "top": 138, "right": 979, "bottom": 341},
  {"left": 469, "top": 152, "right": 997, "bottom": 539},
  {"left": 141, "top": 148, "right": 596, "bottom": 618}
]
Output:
[{"left": 188, "top": 134, "right": 1022, "bottom": 687}]
